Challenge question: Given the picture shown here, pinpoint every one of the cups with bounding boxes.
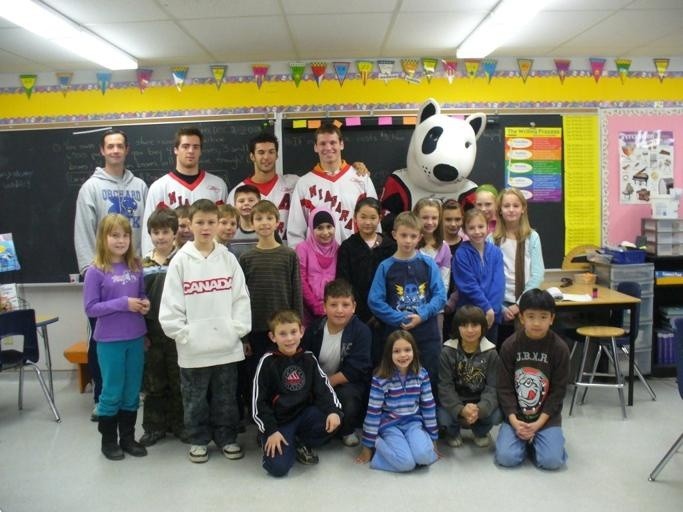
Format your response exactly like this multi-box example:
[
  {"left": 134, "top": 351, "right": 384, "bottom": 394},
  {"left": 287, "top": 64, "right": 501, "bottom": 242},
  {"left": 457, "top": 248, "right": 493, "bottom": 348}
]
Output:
[{"left": 67, "top": 273, "right": 80, "bottom": 284}]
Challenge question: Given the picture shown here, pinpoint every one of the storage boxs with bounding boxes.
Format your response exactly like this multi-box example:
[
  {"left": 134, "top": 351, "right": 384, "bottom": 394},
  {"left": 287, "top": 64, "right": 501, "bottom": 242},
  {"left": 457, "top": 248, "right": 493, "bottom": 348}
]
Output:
[{"left": 654, "top": 330, "right": 673, "bottom": 365}]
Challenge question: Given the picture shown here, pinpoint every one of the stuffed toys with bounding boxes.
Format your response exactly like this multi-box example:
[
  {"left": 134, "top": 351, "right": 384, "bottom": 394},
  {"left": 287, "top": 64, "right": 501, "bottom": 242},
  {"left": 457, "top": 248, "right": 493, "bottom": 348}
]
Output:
[{"left": 377, "top": 96, "right": 486, "bottom": 218}]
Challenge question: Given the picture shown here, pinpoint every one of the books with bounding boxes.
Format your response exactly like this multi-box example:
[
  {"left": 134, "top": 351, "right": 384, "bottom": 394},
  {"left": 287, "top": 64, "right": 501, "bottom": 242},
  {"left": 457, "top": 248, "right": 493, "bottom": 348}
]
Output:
[
  {"left": 668, "top": 332, "right": 676, "bottom": 364},
  {"left": 661, "top": 313, "right": 682, "bottom": 320},
  {"left": 658, "top": 305, "right": 682, "bottom": 316},
  {"left": 653, "top": 328, "right": 662, "bottom": 364},
  {"left": 662, "top": 334, "right": 669, "bottom": 365},
  {"left": 544, "top": 286, "right": 593, "bottom": 303},
  {"left": 660, "top": 320, "right": 682, "bottom": 332}
]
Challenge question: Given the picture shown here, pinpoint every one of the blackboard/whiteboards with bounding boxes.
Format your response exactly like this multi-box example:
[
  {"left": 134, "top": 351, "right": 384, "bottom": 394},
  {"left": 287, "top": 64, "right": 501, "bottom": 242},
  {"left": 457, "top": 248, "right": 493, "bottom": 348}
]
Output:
[
  {"left": 0, "top": 107, "right": 279, "bottom": 288},
  {"left": 277, "top": 108, "right": 602, "bottom": 273}
]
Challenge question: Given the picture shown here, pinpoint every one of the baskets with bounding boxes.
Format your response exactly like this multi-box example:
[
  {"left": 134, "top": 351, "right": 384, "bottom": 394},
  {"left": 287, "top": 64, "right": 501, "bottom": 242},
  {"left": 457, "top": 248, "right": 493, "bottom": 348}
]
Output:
[{"left": 605, "top": 247, "right": 647, "bottom": 264}]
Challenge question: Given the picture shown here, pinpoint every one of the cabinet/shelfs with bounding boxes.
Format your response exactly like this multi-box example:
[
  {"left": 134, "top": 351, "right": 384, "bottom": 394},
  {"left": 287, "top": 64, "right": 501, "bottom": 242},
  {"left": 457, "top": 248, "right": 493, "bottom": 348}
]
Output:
[
  {"left": 653, "top": 272, "right": 682, "bottom": 379},
  {"left": 593, "top": 259, "right": 657, "bottom": 381},
  {"left": 634, "top": 216, "right": 682, "bottom": 261}
]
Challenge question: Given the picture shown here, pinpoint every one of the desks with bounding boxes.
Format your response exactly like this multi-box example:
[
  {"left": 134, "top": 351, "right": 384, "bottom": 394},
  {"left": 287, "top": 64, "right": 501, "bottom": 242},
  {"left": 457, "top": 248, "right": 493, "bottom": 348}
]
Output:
[
  {"left": 29, "top": 312, "right": 62, "bottom": 402},
  {"left": 529, "top": 276, "right": 641, "bottom": 408}
]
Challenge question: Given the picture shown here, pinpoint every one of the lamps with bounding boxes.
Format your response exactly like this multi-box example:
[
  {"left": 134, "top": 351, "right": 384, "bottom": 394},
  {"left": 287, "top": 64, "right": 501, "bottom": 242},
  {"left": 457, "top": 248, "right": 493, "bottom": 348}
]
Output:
[
  {"left": 453, "top": 0, "right": 512, "bottom": 63},
  {"left": 0, "top": 1, "right": 138, "bottom": 77}
]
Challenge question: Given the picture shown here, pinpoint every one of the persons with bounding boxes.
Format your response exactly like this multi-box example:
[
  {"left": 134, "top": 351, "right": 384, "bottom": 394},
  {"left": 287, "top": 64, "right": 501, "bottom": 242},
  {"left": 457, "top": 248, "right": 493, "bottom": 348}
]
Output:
[
  {"left": 84, "top": 184, "right": 568, "bottom": 477},
  {"left": 74, "top": 124, "right": 376, "bottom": 421}
]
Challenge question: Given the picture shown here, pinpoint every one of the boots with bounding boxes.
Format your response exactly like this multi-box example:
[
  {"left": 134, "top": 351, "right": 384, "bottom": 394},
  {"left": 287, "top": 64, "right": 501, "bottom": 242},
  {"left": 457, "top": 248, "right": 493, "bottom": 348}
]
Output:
[
  {"left": 99, "top": 416, "right": 125, "bottom": 460},
  {"left": 118, "top": 409, "right": 147, "bottom": 457}
]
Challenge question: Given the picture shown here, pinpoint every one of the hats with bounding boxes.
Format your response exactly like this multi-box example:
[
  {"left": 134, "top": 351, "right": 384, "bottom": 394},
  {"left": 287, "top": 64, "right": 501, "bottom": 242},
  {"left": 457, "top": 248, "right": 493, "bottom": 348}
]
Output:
[{"left": 313, "top": 211, "right": 335, "bottom": 229}]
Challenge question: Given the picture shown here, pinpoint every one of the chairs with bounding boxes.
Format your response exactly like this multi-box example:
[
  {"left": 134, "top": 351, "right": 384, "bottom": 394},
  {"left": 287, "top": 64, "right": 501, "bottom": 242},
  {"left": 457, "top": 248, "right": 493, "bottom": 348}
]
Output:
[
  {"left": 645, "top": 313, "right": 683, "bottom": 486},
  {"left": 565, "top": 279, "right": 657, "bottom": 413},
  {"left": 0, "top": 306, "right": 61, "bottom": 424}
]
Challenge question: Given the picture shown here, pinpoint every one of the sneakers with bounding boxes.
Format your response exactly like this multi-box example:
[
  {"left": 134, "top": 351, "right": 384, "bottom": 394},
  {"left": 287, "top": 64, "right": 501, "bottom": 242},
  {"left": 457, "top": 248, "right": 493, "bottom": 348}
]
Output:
[
  {"left": 295, "top": 436, "right": 319, "bottom": 465},
  {"left": 471, "top": 432, "right": 490, "bottom": 448},
  {"left": 139, "top": 430, "right": 166, "bottom": 447},
  {"left": 342, "top": 432, "right": 360, "bottom": 446},
  {"left": 189, "top": 444, "right": 209, "bottom": 463},
  {"left": 446, "top": 433, "right": 463, "bottom": 447},
  {"left": 221, "top": 442, "right": 243, "bottom": 459}
]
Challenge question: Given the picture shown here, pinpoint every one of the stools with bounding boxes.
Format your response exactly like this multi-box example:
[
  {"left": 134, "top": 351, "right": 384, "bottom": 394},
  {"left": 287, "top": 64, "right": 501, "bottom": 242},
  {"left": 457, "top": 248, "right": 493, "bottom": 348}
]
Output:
[
  {"left": 570, "top": 324, "right": 631, "bottom": 420},
  {"left": 65, "top": 337, "right": 93, "bottom": 394}
]
